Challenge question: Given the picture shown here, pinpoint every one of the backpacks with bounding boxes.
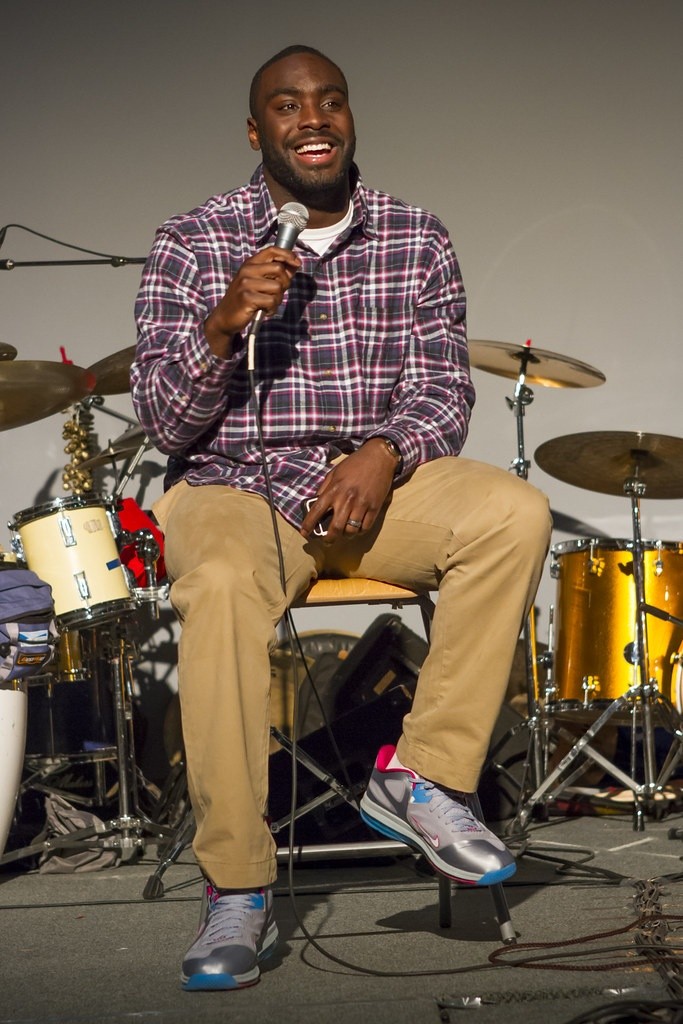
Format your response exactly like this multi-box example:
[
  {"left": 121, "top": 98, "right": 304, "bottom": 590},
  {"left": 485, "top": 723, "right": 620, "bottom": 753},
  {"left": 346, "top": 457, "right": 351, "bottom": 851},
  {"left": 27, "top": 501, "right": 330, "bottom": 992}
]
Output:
[{"left": 0, "top": 553, "right": 59, "bottom": 685}]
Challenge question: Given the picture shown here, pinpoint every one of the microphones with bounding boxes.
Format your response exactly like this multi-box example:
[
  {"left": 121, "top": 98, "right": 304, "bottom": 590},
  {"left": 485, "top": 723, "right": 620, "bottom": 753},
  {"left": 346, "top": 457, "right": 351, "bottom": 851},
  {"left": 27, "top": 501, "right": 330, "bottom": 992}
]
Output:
[{"left": 246, "top": 202, "right": 310, "bottom": 339}]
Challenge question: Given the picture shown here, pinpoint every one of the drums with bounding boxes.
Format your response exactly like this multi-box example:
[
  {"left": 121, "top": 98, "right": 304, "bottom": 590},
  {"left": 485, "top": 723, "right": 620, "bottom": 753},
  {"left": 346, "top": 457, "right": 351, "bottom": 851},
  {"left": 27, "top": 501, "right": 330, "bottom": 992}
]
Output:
[
  {"left": 0, "top": 550, "right": 28, "bottom": 861},
  {"left": 7, "top": 492, "right": 136, "bottom": 633},
  {"left": 24, "top": 629, "right": 96, "bottom": 685},
  {"left": 545, "top": 537, "right": 683, "bottom": 728}
]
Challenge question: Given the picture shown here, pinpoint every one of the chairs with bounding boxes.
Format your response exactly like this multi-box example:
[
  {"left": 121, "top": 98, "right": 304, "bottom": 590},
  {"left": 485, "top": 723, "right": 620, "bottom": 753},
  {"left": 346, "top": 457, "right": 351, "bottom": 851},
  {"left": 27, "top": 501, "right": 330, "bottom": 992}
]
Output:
[{"left": 199, "top": 575, "right": 522, "bottom": 947}]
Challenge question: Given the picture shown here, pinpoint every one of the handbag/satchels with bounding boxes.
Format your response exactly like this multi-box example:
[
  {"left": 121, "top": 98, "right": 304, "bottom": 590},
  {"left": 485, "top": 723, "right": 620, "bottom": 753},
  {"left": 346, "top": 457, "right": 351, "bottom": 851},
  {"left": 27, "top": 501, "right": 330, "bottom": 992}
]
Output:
[{"left": 24, "top": 789, "right": 120, "bottom": 875}]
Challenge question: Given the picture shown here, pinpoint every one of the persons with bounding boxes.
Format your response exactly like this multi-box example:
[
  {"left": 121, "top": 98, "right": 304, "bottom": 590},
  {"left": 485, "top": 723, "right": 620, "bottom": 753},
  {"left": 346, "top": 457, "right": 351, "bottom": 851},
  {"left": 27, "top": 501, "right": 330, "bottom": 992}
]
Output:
[{"left": 131, "top": 45, "right": 554, "bottom": 991}]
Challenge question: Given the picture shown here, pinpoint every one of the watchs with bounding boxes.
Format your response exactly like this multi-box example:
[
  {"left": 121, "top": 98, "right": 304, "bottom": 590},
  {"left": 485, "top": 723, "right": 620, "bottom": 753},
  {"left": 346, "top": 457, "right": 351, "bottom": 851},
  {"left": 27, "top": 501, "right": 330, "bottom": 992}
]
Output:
[{"left": 375, "top": 435, "right": 403, "bottom": 475}]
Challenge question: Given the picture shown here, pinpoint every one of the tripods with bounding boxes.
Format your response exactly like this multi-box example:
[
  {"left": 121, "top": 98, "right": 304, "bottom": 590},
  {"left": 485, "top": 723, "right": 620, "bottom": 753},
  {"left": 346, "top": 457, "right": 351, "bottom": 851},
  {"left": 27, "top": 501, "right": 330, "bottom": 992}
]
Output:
[
  {"left": 0, "top": 624, "right": 182, "bottom": 864},
  {"left": 481, "top": 346, "right": 683, "bottom": 838}
]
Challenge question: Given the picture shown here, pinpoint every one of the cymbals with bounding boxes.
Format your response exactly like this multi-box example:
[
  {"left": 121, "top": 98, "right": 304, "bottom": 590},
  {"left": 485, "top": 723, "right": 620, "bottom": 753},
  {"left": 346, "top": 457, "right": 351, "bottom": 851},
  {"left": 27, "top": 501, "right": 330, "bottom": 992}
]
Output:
[
  {"left": 533, "top": 429, "right": 682, "bottom": 500},
  {"left": 72, "top": 424, "right": 156, "bottom": 469},
  {"left": 84, "top": 343, "right": 137, "bottom": 395},
  {"left": 468, "top": 337, "right": 606, "bottom": 389},
  {"left": 0, "top": 342, "right": 97, "bottom": 432}
]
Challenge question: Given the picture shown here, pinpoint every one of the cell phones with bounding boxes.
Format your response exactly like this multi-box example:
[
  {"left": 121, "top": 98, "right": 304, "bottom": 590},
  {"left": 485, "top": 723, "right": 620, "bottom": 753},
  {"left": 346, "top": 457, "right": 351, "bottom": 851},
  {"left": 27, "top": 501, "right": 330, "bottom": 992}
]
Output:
[{"left": 301, "top": 495, "right": 336, "bottom": 537}]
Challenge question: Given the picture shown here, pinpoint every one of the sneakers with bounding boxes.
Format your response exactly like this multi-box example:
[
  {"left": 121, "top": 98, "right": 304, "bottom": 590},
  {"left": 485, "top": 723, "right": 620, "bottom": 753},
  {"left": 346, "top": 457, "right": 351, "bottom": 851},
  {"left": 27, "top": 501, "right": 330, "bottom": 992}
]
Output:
[
  {"left": 359, "top": 744, "right": 517, "bottom": 885},
  {"left": 179, "top": 886, "right": 280, "bottom": 990}
]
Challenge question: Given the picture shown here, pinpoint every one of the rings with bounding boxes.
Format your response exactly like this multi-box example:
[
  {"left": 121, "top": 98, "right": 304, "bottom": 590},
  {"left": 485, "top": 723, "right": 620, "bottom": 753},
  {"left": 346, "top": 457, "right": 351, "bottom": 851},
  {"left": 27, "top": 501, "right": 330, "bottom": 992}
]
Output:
[{"left": 348, "top": 520, "right": 361, "bottom": 527}]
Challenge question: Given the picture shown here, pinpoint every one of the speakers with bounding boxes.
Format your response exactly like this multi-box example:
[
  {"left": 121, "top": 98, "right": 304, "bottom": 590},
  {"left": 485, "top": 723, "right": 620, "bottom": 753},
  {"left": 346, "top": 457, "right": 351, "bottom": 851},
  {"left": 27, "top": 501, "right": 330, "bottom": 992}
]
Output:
[
  {"left": 328, "top": 612, "right": 559, "bottom": 821},
  {"left": 267, "top": 677, "right": 421, "bottom": 869}
]
